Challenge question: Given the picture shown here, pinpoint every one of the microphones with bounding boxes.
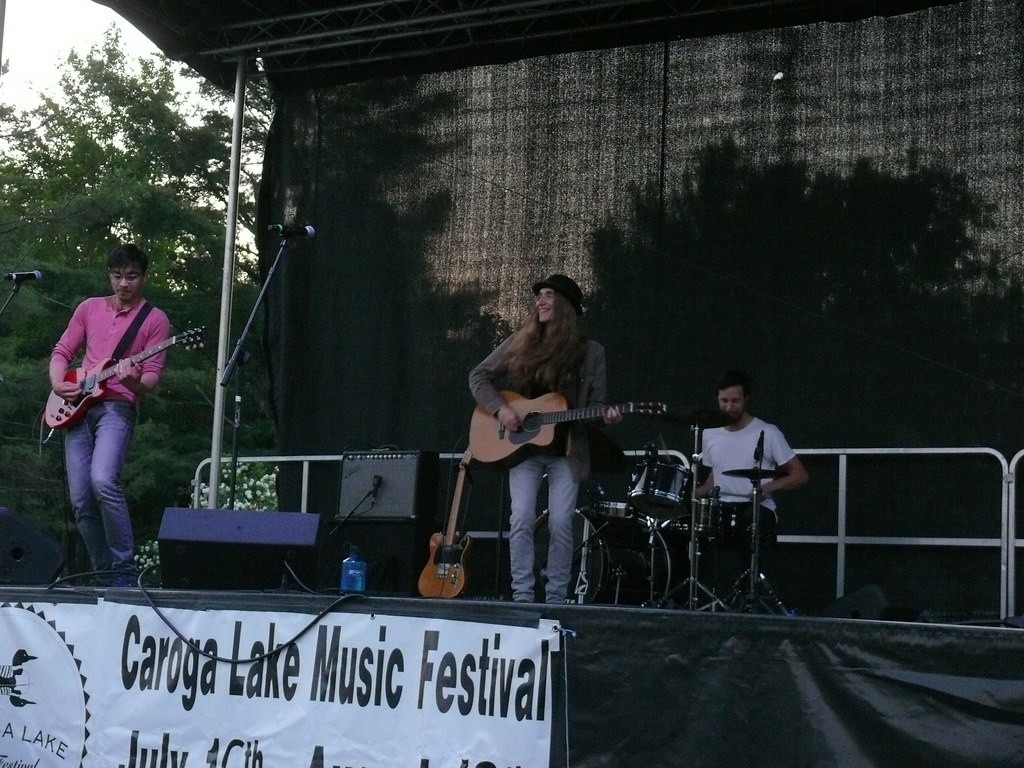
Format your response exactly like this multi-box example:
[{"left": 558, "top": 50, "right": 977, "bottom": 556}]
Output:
[
  {"left": 371, "top": 475, "right": 382, "bottom": 509},
  {"left": 4, "top": 270, "right": 43, "bottom": 281},
  {"left": 267, "top": 225, "right": 315, "bottom": 239},
  {"left": 753, "top": 430, "right": 764, "bottom": 461}
]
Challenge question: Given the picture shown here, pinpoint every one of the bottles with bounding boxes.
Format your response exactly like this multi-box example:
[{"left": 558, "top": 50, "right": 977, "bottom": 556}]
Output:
[{"left": 341, "top": 547, "right": 366, "bottom": 594}]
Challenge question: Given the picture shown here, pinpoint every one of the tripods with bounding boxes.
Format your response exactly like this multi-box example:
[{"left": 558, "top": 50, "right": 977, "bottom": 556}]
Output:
[{"left": 652, "top": 420, "right": 791, "bottom": 618}]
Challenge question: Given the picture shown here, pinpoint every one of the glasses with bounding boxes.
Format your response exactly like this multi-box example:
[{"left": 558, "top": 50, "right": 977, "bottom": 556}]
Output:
[
  {"left": 110, "top": 271, "right": 144, "bottom": 282},
  {"left": 534, "top": 293, "right": 555, "bottom": 301}
]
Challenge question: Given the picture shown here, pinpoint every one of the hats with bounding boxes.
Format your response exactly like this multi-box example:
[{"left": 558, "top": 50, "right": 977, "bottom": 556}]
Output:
[{"left": 533, "top": 274, "right": 584, "bottom": 316}]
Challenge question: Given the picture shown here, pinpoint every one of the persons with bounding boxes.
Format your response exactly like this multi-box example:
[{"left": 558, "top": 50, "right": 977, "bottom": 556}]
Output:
[
  {"left": 49, "top": 244, "right": 170, "bottom": 587},
  {"left": 690, "top": 370, "right": 809, "bottom": 540},
  {"left": 468, "top": 274, "right": 623, "bottom": 603}
]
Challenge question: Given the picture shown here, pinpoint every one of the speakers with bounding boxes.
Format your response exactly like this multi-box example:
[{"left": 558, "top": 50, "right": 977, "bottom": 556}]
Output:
[
  {"left": 336, "top": 450, "right": 440, "bottom": 518},
  {"left": 822, "top": 582, "right": 922, "bottom": 622},
  {"left": 0, "top": 507, "right": 66, "bottom": 585},
  {"left": 158, "top": 506, "right": 328, "bottom": 592}
]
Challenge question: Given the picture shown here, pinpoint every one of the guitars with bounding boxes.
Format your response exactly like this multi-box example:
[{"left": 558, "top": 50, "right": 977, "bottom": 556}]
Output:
[
  {"left": 469, "top": 390, "right": 667, "bottom": 472},
  {"left": 417, "top": 444, "right": 472, "bottom": 599},
  {"left": 45, "top": 326, "right": 206, "bottom": 429}
]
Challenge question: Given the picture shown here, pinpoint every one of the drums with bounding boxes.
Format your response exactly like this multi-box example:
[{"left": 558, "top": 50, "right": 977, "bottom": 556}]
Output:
[
  {"left": 659, "top": 498, "right": 737, "bottom": 544},
  {"left": 533, "top": 499, "right": 671, "bottom": 608},
  {"left": 627, "top": 462, "right": 691, "bottom": 517}
]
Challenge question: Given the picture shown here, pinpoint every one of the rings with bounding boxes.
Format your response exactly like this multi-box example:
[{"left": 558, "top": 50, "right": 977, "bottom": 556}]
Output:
[{"left": 119, "top": 371, "right": 123, "bottom": 373}]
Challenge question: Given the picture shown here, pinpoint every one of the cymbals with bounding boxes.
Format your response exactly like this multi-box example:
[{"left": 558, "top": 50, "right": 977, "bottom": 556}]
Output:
[{"left": 722, "top": 467, "right": 790, "bottom": 480}]
